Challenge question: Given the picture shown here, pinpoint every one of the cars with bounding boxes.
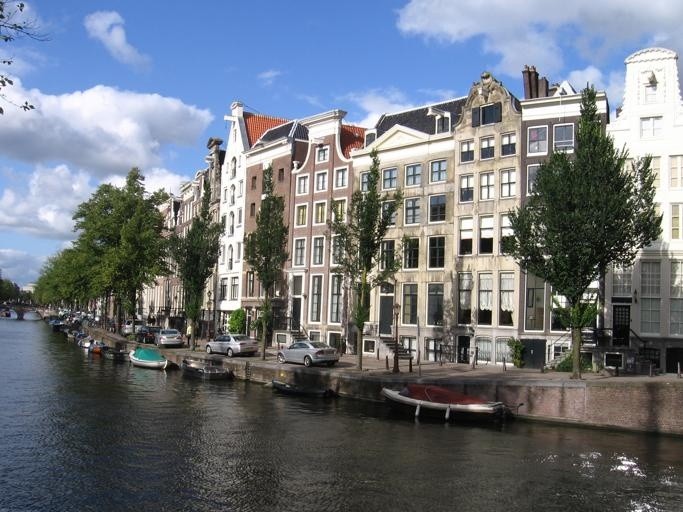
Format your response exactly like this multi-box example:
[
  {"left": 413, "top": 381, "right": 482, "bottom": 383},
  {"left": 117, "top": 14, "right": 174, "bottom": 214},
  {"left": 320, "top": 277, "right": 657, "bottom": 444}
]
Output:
[
  {"left": 153, "top": 328, "right": 184, "bottom": 349},
  {"left": 204, "top": 333, "right": 259, "bottom": 358},
  {"left": 59, "top": 305, "right": 118, "bottom": 334},
  {"left": 277, "top": 340, "right": 340, "bottom": 369}
]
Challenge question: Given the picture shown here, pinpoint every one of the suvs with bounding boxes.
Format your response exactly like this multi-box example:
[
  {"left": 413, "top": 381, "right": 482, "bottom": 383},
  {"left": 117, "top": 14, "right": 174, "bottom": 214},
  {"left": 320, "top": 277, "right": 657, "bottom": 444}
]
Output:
[
  {"left": 120, "top": 319, "right": 144, "bottom": 336},
  {"left": 136, "top": 325, "right": 163, "bottom": 344}
]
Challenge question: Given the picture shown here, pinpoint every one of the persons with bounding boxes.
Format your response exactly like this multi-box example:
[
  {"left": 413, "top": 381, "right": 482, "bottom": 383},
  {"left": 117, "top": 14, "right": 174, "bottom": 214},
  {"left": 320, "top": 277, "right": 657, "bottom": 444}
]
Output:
[
  {"left": 146, "top": 316, "right": 155, "bottom": 326},
  {"left": 187, "top": 323, "right": 199, "bottom": 346}
]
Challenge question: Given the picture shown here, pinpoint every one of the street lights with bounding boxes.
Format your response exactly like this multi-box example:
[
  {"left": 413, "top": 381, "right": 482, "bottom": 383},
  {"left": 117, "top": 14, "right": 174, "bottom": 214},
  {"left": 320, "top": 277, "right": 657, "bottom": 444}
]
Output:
[
  {"left": 207, "top": 290, "right": 213, "bottom": 341},
  {"left": 392, "top": 302, "right": 402, "bottom": 372}
]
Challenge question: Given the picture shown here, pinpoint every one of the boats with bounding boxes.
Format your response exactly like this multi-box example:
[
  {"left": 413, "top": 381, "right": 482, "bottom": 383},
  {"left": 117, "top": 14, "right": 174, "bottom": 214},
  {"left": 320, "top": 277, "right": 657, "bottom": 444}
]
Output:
[
  {"left": 270, "top": 379, "right": 332, "bottom": 399},
  {"left": 377, "top": 384, "right": 511, "bottom": 431},
  {"left": 90, "top": 339, "right": 106, "bottom": 355},
  {"left": 127, "top": 347, "right": 172, "bottom": 369},
  {"left": 99, "top": 346, "right": 132, "bottom": 363},
  {"left": 182, "top": 358, "right": 234, "bottom": 382},
  {"left": 48, "top": 318, "right": 95, "bottom": 349},
  {"left": 1, "top": 308, "right": 10, "bottom": 317}
]
